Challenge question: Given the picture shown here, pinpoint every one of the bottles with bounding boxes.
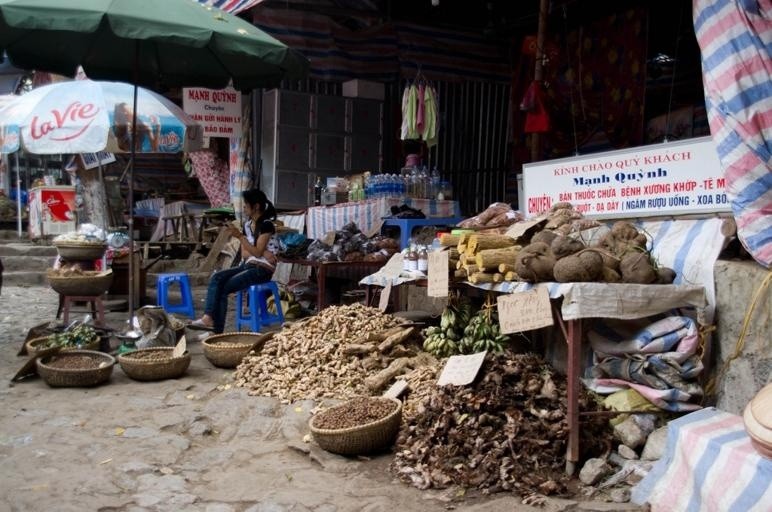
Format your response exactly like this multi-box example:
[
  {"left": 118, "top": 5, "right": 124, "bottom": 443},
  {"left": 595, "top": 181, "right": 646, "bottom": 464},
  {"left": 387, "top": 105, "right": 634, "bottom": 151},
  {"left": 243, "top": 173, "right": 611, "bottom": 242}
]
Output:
[
  {"left": 314, "top": 176, "right": 322, "bottom": 206},
  {"left": 0, "top": 153, "right": 7, "bottom": 194},
  {"left": 404, "top": 244, "right": 429, "bottom": 277},
  {"left": 348, "top": 165, "right": 452, "bottom": 203}
]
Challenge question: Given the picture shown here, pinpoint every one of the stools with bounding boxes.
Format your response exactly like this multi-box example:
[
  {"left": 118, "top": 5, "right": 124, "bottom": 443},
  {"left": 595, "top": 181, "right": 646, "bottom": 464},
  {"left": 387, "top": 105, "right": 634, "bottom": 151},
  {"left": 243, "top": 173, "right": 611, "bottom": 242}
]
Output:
[
  {"left": 158, "top": 270, "right": 195, "bottom": 319},
  {"left": 236, "top": 284, "right": 285, "bottom": 336},
  {"left": 136, "top": 207, "right": 235, "bottom": 260},
  {"left": 55, "top": 292, "right": 103, "bottom": 326}
]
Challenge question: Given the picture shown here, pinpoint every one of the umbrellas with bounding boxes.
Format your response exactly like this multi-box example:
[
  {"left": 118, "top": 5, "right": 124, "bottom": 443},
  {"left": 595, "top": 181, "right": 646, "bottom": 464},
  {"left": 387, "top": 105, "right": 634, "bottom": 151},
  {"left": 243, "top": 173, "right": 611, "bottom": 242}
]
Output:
[
  {"left": 0, "top": 0, "right": 288, "bottom": 330},
  {"left": 0, "top": 62, "right": 202, "bottom": 252}
]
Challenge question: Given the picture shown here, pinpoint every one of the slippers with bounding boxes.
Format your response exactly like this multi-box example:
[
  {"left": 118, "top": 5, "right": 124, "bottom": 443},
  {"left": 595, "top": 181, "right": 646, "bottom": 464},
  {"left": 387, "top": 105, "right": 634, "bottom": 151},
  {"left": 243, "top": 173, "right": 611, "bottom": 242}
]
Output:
[
  {"left": 192, "top": 332, "right": 210, "bottom": 343},
  {"left": 185, "top": 320, "right": 215, "bottom": 330}
]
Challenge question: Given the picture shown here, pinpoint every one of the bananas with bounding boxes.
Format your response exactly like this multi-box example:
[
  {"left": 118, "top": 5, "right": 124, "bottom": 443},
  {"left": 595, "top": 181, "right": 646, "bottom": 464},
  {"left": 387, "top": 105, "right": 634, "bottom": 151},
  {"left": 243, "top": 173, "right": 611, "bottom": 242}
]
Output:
[{"left": 420, "top": 307, "right": 510, "bottom": 357}]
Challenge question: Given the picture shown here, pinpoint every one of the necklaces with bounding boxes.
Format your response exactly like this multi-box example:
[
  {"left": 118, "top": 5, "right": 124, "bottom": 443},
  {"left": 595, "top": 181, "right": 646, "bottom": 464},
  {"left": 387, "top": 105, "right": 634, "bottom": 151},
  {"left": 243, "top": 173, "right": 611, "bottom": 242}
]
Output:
[{"left": 251, "top": 214, "right": 259, "bottom": 220}]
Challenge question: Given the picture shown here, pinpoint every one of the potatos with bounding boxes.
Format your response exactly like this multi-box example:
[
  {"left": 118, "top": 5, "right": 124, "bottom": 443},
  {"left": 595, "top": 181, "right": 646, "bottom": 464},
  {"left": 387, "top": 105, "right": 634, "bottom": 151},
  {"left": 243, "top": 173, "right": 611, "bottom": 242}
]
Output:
[{"left": 516, "top": 221, "right": 677, "bottom": 283}]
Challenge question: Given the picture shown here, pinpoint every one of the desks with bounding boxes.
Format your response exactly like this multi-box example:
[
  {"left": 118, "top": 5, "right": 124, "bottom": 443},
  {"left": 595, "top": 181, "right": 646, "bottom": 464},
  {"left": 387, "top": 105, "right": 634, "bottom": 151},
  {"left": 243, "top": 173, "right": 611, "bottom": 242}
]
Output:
[
  {"left": 253, "top": 256, "right": 390, "bottom": 310},
  {"left": 380, "top": 217, "right": 465, "bottom": 253},
  {"left": 400, "top": 269, "right": 709, "bottom": 473}
]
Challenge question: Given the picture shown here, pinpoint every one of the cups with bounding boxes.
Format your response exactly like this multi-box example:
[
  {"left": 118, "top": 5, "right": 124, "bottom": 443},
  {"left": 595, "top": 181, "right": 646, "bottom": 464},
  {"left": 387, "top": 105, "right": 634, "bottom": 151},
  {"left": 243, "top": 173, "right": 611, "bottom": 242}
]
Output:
[{"left": 43, "top": 175, "right": 56, "bottom": 185}]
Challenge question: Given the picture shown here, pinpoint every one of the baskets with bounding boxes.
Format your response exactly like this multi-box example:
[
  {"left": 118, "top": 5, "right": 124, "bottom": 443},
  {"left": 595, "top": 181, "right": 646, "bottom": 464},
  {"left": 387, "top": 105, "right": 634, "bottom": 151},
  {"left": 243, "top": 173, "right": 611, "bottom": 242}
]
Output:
[
  {"left": 309, "top": 397, "right": 403, "bottom": 456},
  {"left": 202, "top": 332, "right": 264, "bottom": 369},
  {"left": 26, "top": 334, "right": 102, "bottom": 360},
  {"left": 175, "top": 324, "right": 186, "bottom": 338},
  {"left": 55, "top": 245, "right": 107, "bottom": 260},
  {"left": 119, "top": 347, "right": 191, "bottom": 381},
  {"left": 35, "top": 350, "right": 116, "bottom": 388},
  {"left": 49, "top": 271, "right": 114, "bottom": 296}
]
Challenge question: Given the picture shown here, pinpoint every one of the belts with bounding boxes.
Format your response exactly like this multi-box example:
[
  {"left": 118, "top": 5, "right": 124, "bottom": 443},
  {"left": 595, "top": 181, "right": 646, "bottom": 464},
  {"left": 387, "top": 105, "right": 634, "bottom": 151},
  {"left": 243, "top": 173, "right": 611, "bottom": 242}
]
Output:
[{"left": 248, "top": 260, "right": 275, "bottom": 274}]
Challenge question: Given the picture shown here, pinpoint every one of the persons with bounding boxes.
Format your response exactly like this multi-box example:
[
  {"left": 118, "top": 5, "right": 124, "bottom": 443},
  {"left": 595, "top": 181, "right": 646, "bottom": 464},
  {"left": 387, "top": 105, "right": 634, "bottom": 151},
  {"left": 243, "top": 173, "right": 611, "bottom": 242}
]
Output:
[{"left": 187, "top": 190, "right": 280, "bottom": 338}]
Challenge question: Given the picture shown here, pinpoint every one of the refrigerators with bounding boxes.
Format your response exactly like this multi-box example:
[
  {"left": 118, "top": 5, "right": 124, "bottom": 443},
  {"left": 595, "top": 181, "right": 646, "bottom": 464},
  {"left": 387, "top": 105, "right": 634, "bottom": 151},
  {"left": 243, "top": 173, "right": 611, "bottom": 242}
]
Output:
[{"left": 27, "top": 185, "right": 77, "bottom": 240}]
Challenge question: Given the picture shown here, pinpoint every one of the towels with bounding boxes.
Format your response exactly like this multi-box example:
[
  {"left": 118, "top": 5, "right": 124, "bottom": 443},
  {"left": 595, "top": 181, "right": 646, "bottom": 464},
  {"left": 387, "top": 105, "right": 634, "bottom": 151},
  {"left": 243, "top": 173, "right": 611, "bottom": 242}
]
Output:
[{"left": 400, "top": 80, "right": 441, "bottom": 148}]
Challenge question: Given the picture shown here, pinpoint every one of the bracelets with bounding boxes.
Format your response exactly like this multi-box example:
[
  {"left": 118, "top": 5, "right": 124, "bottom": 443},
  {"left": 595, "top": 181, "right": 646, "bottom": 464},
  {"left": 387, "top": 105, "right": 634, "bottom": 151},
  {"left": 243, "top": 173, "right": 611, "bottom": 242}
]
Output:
[{"left": 238, "top": 234, "right": 246, "bottom": 242}]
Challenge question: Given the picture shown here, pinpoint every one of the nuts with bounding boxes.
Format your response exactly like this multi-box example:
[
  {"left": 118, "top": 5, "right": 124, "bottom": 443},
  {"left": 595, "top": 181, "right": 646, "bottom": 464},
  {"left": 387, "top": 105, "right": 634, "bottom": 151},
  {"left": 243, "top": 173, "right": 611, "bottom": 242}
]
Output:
[{"left": 313, "top": 399, "right": 397, "bottom": 429}]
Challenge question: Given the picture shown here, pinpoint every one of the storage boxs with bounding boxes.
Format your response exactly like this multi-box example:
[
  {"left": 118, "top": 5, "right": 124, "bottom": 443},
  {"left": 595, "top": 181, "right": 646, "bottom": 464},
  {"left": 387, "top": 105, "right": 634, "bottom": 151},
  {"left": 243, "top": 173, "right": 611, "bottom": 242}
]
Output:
[{"left": 320, "top": 190, "right": 349, "bottom": 206}]
